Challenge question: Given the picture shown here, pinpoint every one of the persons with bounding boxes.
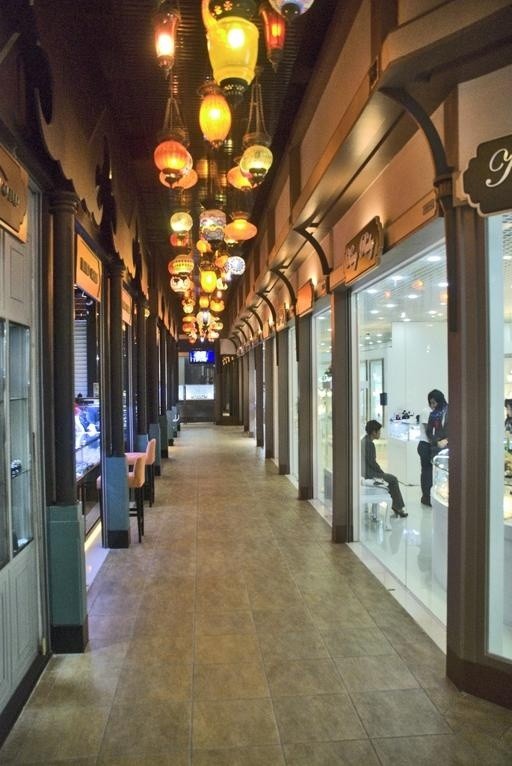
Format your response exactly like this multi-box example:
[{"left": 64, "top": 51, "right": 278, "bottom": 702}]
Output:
[
  {"left": 361, "top": 420, "right": 411, "bottom": 517},
  {"left": 416, "top": 387, "right": 450, "bottom": 448},
  {"left": 505, "top": 398, "right": 511, "bottom": 433}
]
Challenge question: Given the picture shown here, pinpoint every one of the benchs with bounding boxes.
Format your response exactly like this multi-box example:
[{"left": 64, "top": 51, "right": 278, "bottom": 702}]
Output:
[{"left": 324, "top": 466, "right": 393, "bottom": 531}]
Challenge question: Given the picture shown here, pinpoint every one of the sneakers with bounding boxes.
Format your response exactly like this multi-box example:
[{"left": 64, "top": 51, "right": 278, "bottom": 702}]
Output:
[{"left": 421, "top": 496, "right": 431, "bottom": 505}]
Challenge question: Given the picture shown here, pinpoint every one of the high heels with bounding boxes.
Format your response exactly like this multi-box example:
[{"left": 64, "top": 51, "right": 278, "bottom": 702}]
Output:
[{"left": 392, "top": 507, "right": 408, "bottom": 517}]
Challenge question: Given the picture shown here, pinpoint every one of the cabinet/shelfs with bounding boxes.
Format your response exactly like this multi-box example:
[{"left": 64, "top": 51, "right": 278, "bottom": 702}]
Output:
[
  {"left": 75, "top": 431, "right": 101, "bottom": 536},
  {"left": 390, "top": 418, "right": 421, "bottom": 486}
]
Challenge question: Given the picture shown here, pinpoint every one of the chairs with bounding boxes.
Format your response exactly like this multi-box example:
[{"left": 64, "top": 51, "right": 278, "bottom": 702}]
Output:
[
  {"left": 124, "top": 438, "right": 156, "bottom": 507},
  {"left": 96, "top": 454, "right": 146, "bottom": 543}
]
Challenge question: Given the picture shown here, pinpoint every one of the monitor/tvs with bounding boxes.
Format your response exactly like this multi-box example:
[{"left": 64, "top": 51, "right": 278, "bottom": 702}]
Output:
[{"left": 189, "top": 350, "right": 209, "bottom": 364}]
